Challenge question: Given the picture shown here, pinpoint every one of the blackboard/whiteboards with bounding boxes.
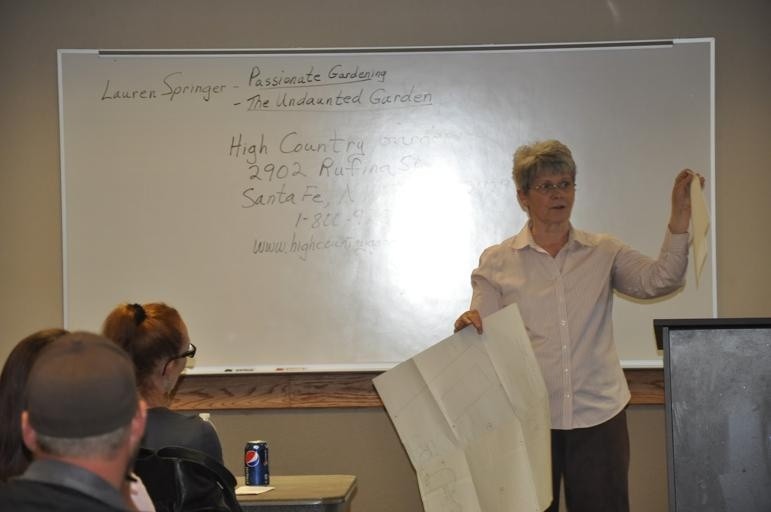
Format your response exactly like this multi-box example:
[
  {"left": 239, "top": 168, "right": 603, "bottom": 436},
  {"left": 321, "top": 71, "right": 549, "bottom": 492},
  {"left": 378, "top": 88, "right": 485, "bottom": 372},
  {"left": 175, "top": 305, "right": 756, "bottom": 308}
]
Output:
[{"left": 56, "top": 37, "right": 718, "bottom": 375}]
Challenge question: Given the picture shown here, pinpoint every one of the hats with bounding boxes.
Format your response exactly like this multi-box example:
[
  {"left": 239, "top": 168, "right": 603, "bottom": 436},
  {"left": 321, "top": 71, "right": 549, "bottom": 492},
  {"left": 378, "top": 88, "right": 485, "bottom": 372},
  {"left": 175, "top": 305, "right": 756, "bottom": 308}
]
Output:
[{"left": 27, "top": 331, "right": 139, "bottom": 437}]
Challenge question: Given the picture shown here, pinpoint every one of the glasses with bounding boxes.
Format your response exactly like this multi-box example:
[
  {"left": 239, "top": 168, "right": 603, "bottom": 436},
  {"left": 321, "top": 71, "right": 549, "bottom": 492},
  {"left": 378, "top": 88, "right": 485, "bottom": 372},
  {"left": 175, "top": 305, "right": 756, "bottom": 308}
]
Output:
[
  {"left": 163, "top": 343, "right": 196, "bottom": 376},
  {"left": 525, "top": 179, "right": 573, "bottom": 194}
]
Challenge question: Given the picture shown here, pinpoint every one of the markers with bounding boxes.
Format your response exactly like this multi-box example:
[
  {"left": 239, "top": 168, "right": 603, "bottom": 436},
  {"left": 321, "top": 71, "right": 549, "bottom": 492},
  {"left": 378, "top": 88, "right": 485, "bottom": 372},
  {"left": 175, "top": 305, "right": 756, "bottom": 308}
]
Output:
[
  {"left": 184, "top": 365, "right": 194, "bottom": 370},
  {"left": 276, "top": 367, "right": 304, "bottom": 371},
  {"left": 224, "top": 368, "right": 253, "bottom": 372}
]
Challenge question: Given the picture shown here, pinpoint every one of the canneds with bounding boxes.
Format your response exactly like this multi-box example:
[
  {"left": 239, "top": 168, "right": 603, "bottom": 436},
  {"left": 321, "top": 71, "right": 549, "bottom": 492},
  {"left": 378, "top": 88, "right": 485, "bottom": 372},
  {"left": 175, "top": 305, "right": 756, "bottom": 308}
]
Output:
[{"left": 243, "top": 439, "right": 270, "bottom": 486}]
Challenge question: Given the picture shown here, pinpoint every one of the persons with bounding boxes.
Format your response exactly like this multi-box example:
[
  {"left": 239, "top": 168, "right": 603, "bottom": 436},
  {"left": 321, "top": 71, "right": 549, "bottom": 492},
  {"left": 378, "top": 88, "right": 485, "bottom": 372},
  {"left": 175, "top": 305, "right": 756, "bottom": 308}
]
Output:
[
  {"left": 101, "top": 301, "right": 232, "bottom": 511},
  {"left": 453, "top": 139, "right": 704, "bottom": 510},
  {"left": 2, "top": 330, "right": 150, "bottom": 510},
  {"left": 0, "top": 328, "right": 75, "bottom": 479}
]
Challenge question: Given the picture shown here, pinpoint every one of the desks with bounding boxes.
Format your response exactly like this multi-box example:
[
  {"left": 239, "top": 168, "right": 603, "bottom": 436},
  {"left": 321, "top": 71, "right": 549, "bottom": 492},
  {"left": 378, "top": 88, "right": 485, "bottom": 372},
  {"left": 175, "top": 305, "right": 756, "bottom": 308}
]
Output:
[{"left": 231, "top": 473, "right": 361, "bottom": 512}]
